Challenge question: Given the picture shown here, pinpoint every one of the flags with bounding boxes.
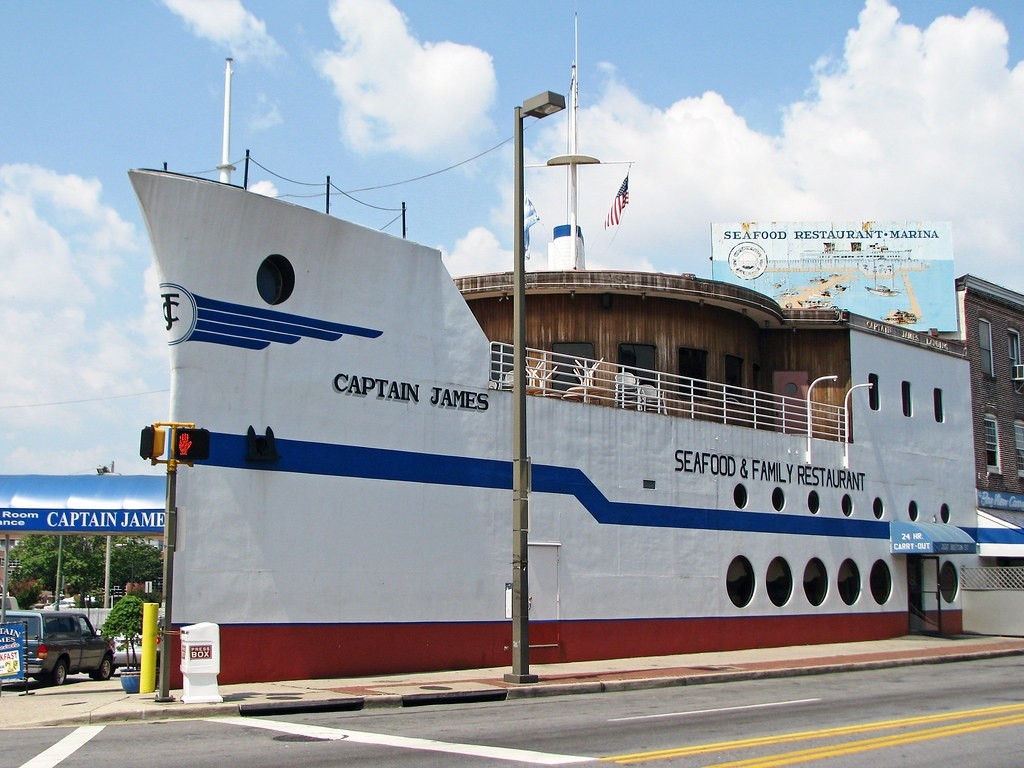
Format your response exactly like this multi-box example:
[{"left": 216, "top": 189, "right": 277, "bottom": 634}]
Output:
[
  {"left": 524, "top": 197, "right": 540, "bottom": 260},
  {"left": 604, "top": 172, "right": 628, "bottom": 230}
]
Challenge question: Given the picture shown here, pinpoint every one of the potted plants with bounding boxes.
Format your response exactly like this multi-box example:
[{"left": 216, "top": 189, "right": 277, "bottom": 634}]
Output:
[{"left": 101, "top": 595, "right": 144, "bottom": 694}]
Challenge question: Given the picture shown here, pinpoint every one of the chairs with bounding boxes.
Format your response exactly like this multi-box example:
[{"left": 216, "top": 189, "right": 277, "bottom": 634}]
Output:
[
  {"left": 502, "top": 370, "right": 514, "bottom": 388},
  {"left": 615, "top": 371, "right": 640, "bottom": 412},
  {"left": 640, "top": 384, "right": 668, "bottom": 416},
  {"left": 489, "top": 381, "right": 498, "bottom": 389}
]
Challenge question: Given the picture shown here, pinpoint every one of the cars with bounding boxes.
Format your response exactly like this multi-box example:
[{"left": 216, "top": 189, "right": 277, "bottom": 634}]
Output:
[
  {"left": 44, "top": 595, "right": 124, "bottom": 631},
  {"left": 1, "top": 609, "right": 114, "bottom": 687},
  {"left": 100, "top": 633, "right": 144, "bottom": 671}
]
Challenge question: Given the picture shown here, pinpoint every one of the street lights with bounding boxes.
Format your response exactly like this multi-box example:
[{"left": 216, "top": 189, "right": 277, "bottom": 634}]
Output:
[{"left": 500, "top": 91, "right": 567, "bottom": 686}]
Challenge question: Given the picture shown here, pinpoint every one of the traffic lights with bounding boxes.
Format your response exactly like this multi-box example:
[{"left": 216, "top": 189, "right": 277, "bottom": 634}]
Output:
[
  {"left": 139, "top": 426, "right": 165, "bottom": 460},
  {"left": 174, "top": 425, "right": 211, "bottom": 461}
]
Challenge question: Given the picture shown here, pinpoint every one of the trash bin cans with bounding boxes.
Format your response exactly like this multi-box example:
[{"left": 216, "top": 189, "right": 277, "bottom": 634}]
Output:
[{"left": 179, "top": 621, "right": 224, "bottom": 703}]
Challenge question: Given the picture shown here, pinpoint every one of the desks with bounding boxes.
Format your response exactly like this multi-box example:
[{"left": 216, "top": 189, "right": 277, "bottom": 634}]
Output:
[{"left": 566, "top": 387, "right": 607, "bottom": 406}]
